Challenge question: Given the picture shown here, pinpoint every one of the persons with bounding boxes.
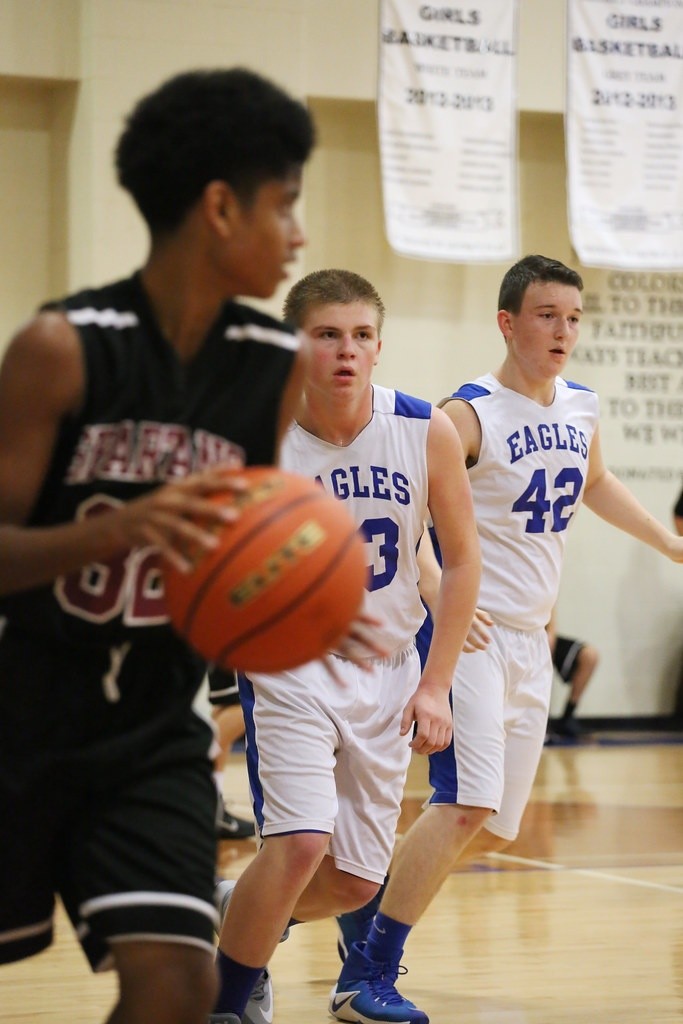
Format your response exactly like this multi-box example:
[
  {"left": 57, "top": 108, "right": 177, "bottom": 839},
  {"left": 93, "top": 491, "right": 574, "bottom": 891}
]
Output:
[
  {"left": 330, "top": 253, "right": 683, "bottom": 1024},
  {"left": 546, "top": 610, "right": 599, "bottom": 739},
  {"left": 214, "top": 270, "right": 483, "bottom": 1024},
  {"left": 0, "top": 66, "right": 389, "bottom": 1024},
  {"left": 192, "top": 658, "right": 255, "bottom": 841}
]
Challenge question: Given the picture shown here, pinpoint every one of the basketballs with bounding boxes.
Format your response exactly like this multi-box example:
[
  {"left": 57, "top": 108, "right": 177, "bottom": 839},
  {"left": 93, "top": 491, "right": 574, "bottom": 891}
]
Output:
[{"left": 159, "top": 462, "right": 368, "bottom": 675}]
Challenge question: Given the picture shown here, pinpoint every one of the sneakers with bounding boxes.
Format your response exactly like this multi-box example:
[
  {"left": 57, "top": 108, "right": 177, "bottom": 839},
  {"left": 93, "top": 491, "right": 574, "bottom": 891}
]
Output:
[
  {"left": 328, "top": 910, "right": 429, "bottom": 1024},
  {"left": 210, "top": 877, "right": 290, "bottom": 1023}
]
